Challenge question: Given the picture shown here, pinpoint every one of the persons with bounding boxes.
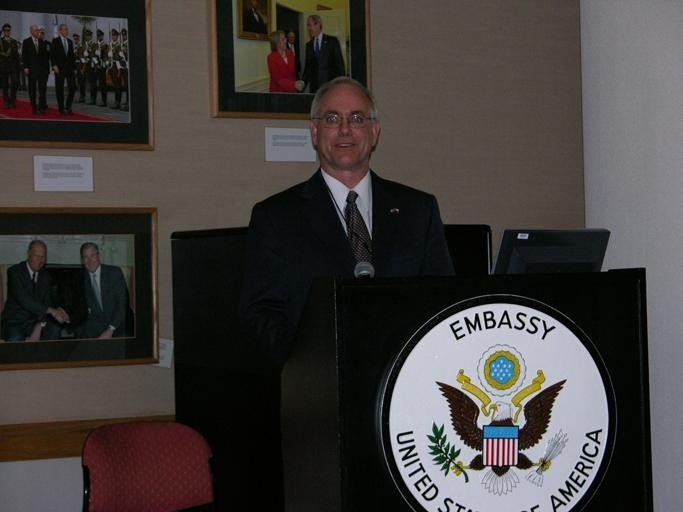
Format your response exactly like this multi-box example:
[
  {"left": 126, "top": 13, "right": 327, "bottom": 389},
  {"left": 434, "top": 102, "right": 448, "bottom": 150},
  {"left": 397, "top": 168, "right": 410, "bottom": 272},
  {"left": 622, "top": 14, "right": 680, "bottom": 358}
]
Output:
[
  {"left": 58, "top": 241, "right": 130, "bottom": 338},
  {"left": 0, "top": 23, "right": 128, "bottom": 115},
  {"left": 243, "top": 0, "right": 343, "bottom": 94},
  {"left": 1, "top": 239, "right": 65, "bottom": 343},
  {"left": 238, "top": 75, "right": 455, "bottom": 377}
]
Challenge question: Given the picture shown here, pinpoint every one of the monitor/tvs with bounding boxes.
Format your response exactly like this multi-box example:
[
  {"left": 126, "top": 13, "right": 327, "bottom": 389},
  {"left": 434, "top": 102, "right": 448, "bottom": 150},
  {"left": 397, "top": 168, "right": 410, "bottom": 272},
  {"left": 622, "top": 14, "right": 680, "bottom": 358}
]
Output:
[{"left": 494, "top": 226, "right": 611, "bottom": 274}]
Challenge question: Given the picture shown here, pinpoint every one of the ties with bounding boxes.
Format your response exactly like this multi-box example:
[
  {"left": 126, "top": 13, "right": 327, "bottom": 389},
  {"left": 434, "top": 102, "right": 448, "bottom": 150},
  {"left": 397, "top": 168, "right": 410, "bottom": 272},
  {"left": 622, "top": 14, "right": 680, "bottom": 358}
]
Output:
[
  {"left": 91, "top": 271, "right": 100, "bottom": 302},
  {"left": 315, "top": 38, "right": 320, "bottom": 52},
  {"left": 31, "top": 273, "right": 39, "bottom": 280},
  {"left": 345, "top": 191, "right": 373, "bottom": 262},
  {"left": 63, "top": 39, "right": 68, "bottom": 52}
]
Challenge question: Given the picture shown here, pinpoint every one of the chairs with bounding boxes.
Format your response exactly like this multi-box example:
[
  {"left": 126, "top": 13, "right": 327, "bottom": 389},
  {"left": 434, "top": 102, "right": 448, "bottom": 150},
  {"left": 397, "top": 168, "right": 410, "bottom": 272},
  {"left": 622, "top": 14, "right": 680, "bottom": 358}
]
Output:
[{"left": 82, "top": 423, "right": 213, "bottom": 511}]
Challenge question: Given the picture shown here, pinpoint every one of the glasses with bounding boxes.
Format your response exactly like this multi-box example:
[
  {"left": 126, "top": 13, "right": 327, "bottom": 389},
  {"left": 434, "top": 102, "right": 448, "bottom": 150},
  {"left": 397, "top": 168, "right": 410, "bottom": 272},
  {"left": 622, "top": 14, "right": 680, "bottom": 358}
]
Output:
[
  {"left": 307, "top": 23, "right": 317, "bottom": 27},
  {"left": 313, "top": 111, "right": 376, "bottom": 128}
]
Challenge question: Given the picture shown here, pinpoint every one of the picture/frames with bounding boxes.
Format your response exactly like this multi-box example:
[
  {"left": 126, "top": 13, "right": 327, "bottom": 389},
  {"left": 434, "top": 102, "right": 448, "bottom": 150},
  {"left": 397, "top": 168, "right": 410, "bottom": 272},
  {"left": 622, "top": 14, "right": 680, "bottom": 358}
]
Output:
[
  {"left": 0, "top": 207, "right": 160, "bottom": 371},
  {"left": 207, "top": 0, "right": 373, "bottom": 120},
  {"left": 0, "top": 0, "right": 156, "bottom": 151}
]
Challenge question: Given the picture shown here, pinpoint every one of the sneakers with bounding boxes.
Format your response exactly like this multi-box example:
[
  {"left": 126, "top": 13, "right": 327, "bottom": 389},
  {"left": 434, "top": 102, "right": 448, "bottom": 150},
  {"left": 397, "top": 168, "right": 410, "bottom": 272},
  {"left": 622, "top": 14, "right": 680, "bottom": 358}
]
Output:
[{"left": 1, "top": 98, "right": 129, "bottom": 116}]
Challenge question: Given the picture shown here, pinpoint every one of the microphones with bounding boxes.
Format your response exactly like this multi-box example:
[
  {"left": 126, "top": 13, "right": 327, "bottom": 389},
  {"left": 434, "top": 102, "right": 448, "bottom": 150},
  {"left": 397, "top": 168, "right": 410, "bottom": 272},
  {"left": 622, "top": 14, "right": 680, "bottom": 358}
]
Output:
[{"left": 354, "top": 261, "right": 375, "bottom": 278}]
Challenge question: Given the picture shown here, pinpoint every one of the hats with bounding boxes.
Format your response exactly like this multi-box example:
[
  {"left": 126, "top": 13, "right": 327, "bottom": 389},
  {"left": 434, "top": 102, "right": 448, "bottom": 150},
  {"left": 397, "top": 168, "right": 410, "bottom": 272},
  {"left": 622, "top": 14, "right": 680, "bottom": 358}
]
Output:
[
  {"left": 97, "top": 30, "right": 104, "bottom": 35},
  {"left": 121, "top": 28, "right": 127, "bottom": 35},
  {"left": 38, "top": 25, "right": 45, "bottom": 30},
  {"left": 112, "top": 28, "right": 120, "bottom": 36},
  {"left": 85, "top": 30, "right": 92, "bottom": 35},
  {"left": 73, "top": 34, "right": 80, "bottom": 38},
  {"left": 3, "top": 23, "right": 11, "bottom": 32}
]
